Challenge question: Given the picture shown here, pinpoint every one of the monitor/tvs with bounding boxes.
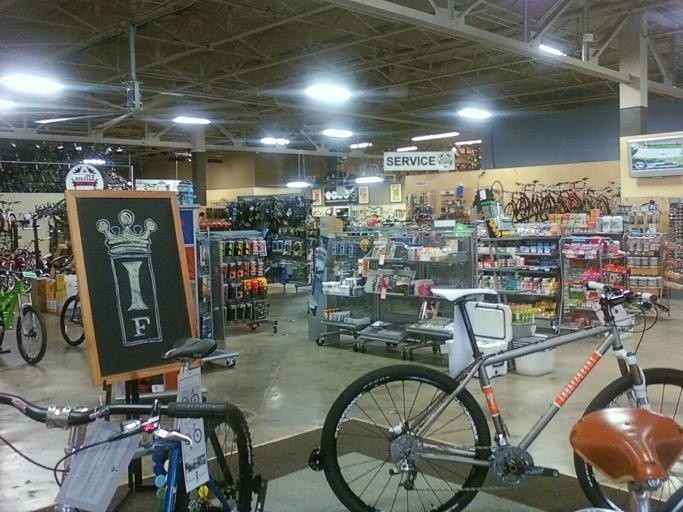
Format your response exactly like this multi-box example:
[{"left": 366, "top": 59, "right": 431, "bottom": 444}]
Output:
[{"left": 626, "top": 134, "right": 683, "bottom": 178}]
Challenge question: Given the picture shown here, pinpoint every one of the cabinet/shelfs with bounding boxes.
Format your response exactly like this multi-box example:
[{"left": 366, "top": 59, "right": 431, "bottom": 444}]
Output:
[{"left": 313, "top": 221, "right": 665, "bottom": 378}]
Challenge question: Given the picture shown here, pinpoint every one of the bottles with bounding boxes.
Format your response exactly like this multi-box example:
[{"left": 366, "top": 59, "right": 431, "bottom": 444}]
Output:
[{"left": 506, "top": 301, "right": 533, "bottom": 324}]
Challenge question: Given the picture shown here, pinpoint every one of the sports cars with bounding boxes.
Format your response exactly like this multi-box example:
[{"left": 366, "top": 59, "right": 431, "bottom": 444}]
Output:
[{"left": 631, "top": 158, "right": 682, "bottom": 169}]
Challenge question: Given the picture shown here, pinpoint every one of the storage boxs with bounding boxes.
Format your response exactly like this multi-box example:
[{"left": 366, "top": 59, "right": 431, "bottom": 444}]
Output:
[{"left": 26, "top": 274, "right": 65, "bottom": 316}]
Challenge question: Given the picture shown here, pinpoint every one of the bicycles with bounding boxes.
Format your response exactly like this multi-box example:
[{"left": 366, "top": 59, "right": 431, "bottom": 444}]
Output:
[
  {"left": 0, "top": 335, "right": 268, "bottom": 510},
  {"left": 0, "top": 198, "right": 87, "bottom": 365},
  {"left": 0, "top": 140, "right": 126, "bottom": 192},
  {"left": 308, "top": 279, "right": 683, "bottom": 512},
  {"left": 359, "top": 139, "right": 481, "bottom": 173},
  {"left": 563, "top": 408, "right": 683, "bottom": 511},
  {"left": 477, "top": 169, "right": 621, "bottom": 224}
]
